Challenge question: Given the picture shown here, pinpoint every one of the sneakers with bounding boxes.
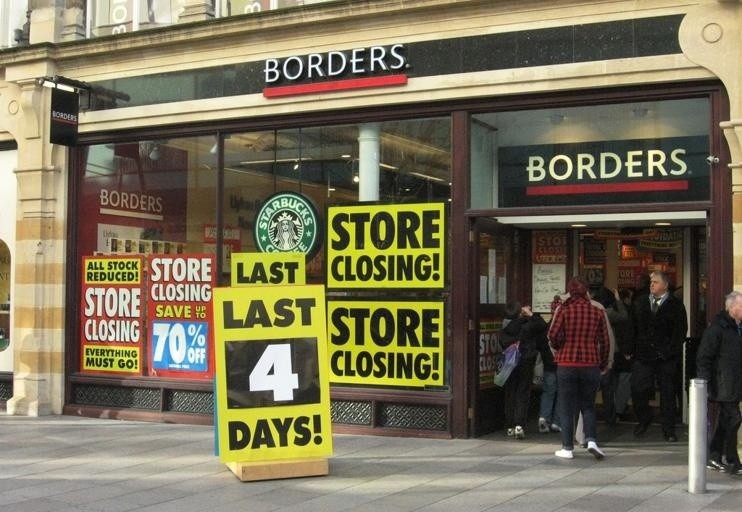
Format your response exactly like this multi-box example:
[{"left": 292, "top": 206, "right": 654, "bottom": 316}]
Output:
[
  {"left": 555, "top": 449, "right": 573, "bottom": 459},
  {"left": 588, "top": 441, "right": 605, "bottom": 459},
  {"left": 507, "top": 426, "right": 525, "bottom": 439},
  {"left": 539, "top": 417, "right": 562, "bottom": 433}
]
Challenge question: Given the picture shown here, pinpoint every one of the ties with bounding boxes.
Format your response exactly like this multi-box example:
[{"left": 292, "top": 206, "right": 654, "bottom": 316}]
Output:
[{"left": 653, "top": 297, "right": 661, "bottom": 312}]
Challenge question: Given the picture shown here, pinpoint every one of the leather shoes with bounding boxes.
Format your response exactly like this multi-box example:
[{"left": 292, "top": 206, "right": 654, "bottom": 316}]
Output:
[
  {"left": 706, "top": 459, "right": 742, "bottom": 475},
  {"left": 634, "top": 424, "right": 648, "bottom": 435},
  {"left": 665, "top": 432, "right": 677, "bottom": 442}
]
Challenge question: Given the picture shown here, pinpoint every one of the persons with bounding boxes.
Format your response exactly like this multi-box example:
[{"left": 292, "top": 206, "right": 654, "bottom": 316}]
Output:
[
  {"left": 537, "top": 270, "right": 688, "bottom": 441},
  {"left": 501, "top": 297, "right": 542, "bottom": 438},
  {"left": 695, "top": 290, "right": 742, "bottom": 475},
  {"left": 548, "top": 275, "right": 610, "bottom": 459}
]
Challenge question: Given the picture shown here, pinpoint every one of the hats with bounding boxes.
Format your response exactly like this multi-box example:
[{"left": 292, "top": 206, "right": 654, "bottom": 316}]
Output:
[{"left": 568, "top": 276, "right": 589, "bottom": 296}]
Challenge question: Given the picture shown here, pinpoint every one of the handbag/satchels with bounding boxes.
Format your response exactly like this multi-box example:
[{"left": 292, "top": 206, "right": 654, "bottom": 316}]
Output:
[
  {"left": 533, "top": 348, "right": 556, "bottom": 386},
  {"left": 494, "top": 344, "right": 521, "bottom": 387}
]
